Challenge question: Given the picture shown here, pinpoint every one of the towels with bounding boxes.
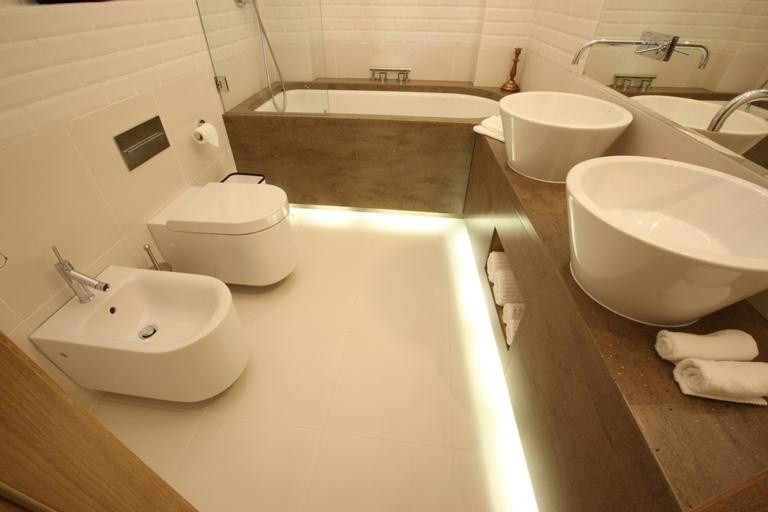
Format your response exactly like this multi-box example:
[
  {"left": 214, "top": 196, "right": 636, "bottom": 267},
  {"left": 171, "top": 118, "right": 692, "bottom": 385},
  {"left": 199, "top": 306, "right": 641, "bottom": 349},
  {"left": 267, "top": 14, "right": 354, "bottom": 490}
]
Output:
[
  {"left": 654, "top": 327, "right": 768, "bottom": 405},
  {"left": 486, "top": 251, "right": 526, "bottom": 347},
  {"left": 473, "top": 115, "right": 506, "bottom": 144}
]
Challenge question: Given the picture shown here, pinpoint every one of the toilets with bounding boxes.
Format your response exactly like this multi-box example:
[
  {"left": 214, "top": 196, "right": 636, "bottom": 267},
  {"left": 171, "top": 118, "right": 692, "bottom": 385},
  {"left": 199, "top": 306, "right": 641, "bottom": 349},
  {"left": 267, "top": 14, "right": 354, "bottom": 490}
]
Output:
[{"left": 148, "top": 180, "right": 298, "bottom": 286}]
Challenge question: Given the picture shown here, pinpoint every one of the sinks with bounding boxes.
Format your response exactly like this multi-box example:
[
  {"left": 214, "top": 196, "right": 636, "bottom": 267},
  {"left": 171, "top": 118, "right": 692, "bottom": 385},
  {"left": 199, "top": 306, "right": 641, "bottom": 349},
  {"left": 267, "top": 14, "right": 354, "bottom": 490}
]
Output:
[
  {"left": 564, "top": 155, "right": 768, "bottom": 328},
  {"left": 498, "top": 90, "right": 632, "bottom": 185},
  {"left": 631, "top": 93, "right": 768, "bottom": 156},
  {"left": 29, "top": 265, "right": 248, "bottom": 403}
]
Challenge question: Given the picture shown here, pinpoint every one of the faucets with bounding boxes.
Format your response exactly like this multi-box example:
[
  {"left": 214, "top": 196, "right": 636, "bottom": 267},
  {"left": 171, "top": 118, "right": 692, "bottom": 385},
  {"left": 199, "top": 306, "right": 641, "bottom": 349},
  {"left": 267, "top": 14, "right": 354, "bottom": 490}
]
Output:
[
  {"left": 673, "top": 39, "right": 711, "bottom": 71},
  {"left": 51, "top": 244, "right": 110, "bottom": 303},
  {"left": 703, "top": 86, "right": 767, "bottom": 131},
  {"left": 571, "top": 37, "right": 664, "bottom": 67}
]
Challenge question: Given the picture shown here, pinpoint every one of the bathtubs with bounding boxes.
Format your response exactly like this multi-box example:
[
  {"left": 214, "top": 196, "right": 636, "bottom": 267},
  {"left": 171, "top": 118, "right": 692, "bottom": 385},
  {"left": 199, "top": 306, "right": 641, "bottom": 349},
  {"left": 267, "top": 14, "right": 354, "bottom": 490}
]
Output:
[{"left": 243, "top": 80, "right": 500, "bottom": 122}]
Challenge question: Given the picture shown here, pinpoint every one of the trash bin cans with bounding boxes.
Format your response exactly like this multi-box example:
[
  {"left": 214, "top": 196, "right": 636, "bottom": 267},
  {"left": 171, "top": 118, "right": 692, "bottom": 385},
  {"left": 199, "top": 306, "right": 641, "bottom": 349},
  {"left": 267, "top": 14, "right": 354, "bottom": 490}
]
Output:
[{"left": 220, "top": 172, "right": 267, "bottom": 183}]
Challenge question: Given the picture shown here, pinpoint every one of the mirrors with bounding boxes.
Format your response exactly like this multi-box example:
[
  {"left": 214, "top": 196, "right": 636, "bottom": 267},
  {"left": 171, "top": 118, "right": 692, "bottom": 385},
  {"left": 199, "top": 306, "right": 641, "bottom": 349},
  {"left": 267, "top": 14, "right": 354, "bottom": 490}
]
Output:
[{"left": 581, "top": 1, "right": 768, "bottom": 181}]
